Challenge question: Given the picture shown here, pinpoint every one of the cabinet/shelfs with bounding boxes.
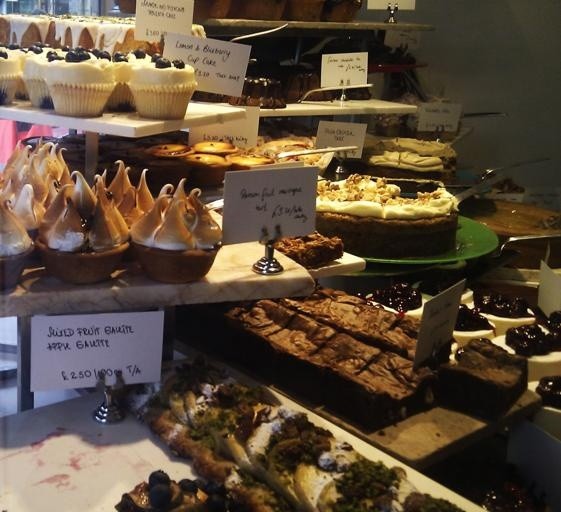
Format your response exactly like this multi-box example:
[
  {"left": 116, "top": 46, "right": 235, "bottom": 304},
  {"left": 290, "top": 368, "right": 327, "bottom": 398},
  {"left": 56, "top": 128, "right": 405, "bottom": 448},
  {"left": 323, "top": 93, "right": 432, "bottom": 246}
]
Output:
[{"left": 2, "top": 0, "right": 561, "bottom": 512}]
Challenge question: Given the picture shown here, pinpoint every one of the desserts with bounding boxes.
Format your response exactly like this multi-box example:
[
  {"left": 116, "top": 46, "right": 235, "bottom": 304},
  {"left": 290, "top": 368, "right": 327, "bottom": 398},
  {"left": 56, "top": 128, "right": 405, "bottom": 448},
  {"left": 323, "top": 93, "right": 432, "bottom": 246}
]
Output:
[{"left": 0, "top": 13, "right": 561, "bottom": 512}]
